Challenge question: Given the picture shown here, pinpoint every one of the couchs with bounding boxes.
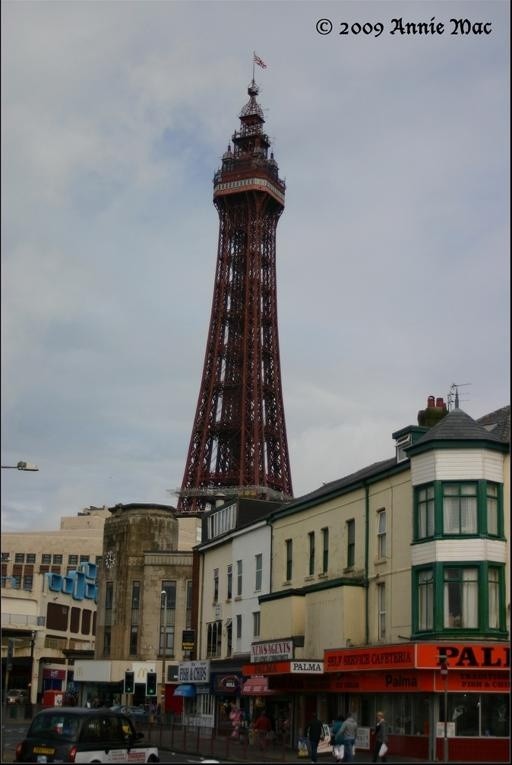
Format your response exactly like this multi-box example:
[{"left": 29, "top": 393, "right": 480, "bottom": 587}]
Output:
[
  {"left": 125, "top": 671, "right": 136, "bottom": 695},
  {"left": 145, "top": 672, "right": 159, "bottom": 697}
]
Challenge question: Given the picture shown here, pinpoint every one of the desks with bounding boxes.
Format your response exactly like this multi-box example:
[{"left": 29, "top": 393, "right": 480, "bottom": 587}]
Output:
[
  {"left": 159, "top": 589, "right": 168, "bottom": 723},
  {"left": 1, "top": 461, "right": 39, "bottom": 473}
]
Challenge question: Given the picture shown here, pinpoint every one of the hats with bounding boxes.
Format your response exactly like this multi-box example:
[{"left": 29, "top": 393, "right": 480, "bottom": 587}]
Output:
[{"left": 6, "top": 689, "right": 30, "bottom": 705}]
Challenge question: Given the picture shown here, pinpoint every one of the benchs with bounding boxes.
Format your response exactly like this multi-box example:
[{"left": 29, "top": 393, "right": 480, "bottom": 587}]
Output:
[{"left": 255, "top": 55, "right": 267, "bottom": 70}]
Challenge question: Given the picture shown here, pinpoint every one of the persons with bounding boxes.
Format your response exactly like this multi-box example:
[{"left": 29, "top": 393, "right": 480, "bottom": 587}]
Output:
[
  {"left": 332, "top": 715, "right": 345, "bottom": 734},
  {"left": 371, "top": 712, "right": 387, "bottom": 763},
  {"left": 334, "top": 713, "right": 358, "bottom": 762},
  {"left": 304, "top": 712, "right": 325, "bottom": 762},
  {"left": 254, "top": 710, "right": 272, "bottom": 752}
]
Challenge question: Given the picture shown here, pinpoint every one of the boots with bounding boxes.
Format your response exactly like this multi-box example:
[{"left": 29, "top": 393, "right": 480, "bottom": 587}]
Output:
[{"left": 12, "top": 706, "right": 162, "bottom": 764}]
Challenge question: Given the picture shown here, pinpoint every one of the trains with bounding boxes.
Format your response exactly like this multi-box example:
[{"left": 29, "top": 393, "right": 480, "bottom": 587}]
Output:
[{"left": 440, "top": 664, "right": 447, "bottom": 680}]
